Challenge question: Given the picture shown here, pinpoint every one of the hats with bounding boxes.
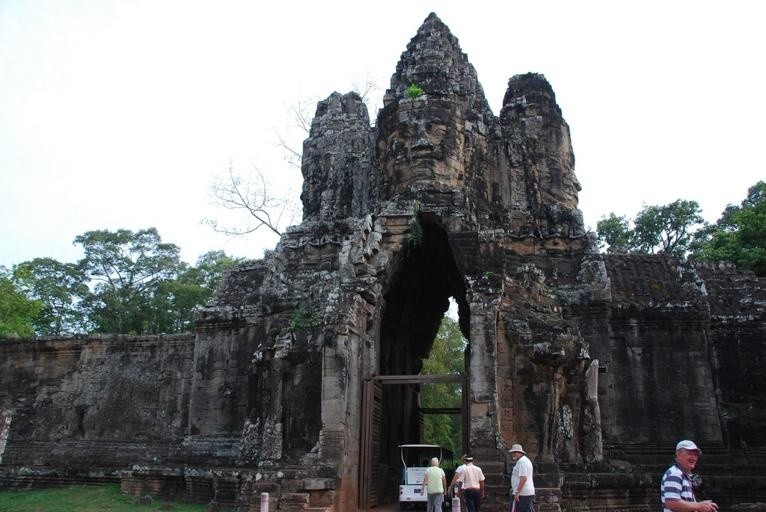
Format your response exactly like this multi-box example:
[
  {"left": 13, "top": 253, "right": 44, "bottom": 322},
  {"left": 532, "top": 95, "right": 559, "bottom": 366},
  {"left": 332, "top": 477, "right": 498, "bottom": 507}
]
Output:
[
  {"left": 674, "top": 439, "right": 703, "bottom": 458},
  {"left": 507, "top": 444, "right": 526, "bottom": 455}
]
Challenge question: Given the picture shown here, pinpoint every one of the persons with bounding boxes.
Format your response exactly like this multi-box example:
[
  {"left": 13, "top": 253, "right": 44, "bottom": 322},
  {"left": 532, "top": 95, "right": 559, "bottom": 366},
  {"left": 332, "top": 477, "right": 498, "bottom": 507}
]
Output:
[
  {"left": 508, "top": 444, "right": 535, "bottom": 512},
  {"left": 661, "top": 438, "right": 721, "bottom": 512},
  {"left": 423, "top": 456, "right": 486, "bottom": 512}
]
